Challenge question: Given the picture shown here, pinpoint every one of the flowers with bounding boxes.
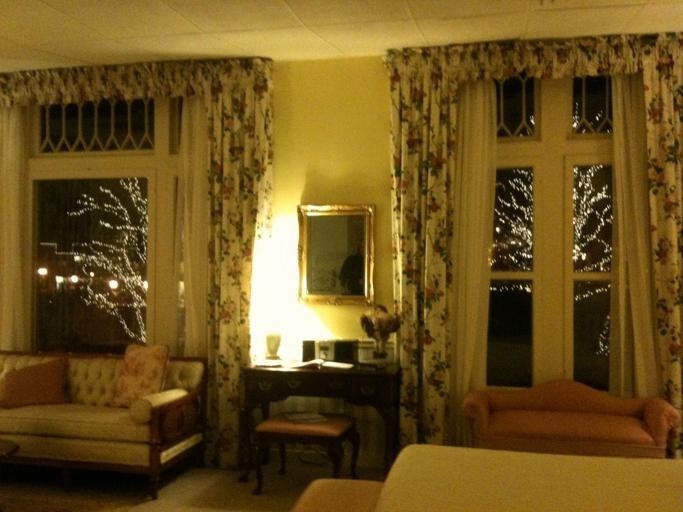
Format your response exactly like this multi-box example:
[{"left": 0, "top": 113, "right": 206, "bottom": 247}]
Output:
[{"left": 360, "top": 303, "right": 401, "bottom": 340}]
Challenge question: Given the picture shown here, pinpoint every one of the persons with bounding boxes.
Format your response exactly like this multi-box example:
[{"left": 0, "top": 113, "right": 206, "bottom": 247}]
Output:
[{"left": 340, "top": 239, "right": 365, "bottom": 294}]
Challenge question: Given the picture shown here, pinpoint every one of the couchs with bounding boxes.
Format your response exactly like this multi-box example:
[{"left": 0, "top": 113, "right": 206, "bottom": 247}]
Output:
[
  {"left": 0, "top": 350, "right": 208, "bottom": 500},
  {"left": 460, "top": 378, "right": 682, "bottom": 459}
]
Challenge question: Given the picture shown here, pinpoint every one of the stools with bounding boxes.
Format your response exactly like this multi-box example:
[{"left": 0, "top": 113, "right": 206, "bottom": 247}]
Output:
[{"left": 251, "top": 412, "right": 359, "bottom": 496}]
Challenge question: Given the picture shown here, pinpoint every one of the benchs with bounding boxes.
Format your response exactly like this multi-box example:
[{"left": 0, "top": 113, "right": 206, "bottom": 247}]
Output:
[{"left": 292, "top": 478, "right": 384, "bottom": 512}]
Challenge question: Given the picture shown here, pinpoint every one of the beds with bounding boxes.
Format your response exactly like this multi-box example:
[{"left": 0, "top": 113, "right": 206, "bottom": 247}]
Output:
[{"left": 375, "top": 443, "right": 683, "bottom": 512}]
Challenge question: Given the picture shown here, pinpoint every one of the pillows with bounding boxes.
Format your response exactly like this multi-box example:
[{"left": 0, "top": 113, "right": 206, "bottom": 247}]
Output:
[
  {"left": 109, "top": 344, "right": 169, "bottom": 408},
  {"left": 1, "top": 358, "right": 64, "bottom": 407}
]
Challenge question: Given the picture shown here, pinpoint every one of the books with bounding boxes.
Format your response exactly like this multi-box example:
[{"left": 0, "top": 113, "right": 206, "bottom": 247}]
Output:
[{"left": 293, "top": 359, "right": 354, "bottom": 374}]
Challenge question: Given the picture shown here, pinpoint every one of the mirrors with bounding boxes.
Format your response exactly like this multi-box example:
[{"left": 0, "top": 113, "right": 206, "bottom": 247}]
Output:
[{"left": 297, "top": 204, "right": 375, "bottom": 308}]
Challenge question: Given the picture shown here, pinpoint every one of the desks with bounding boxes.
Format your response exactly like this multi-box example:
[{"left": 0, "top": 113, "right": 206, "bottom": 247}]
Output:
[{"left": 239, "top": 361, "right": 401, "bottom": 481}]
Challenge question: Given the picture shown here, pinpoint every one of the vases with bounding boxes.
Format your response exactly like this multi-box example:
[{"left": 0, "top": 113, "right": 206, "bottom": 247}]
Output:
[{"left": 371, "top": 336, "right": 388, "bottom": 370}]
